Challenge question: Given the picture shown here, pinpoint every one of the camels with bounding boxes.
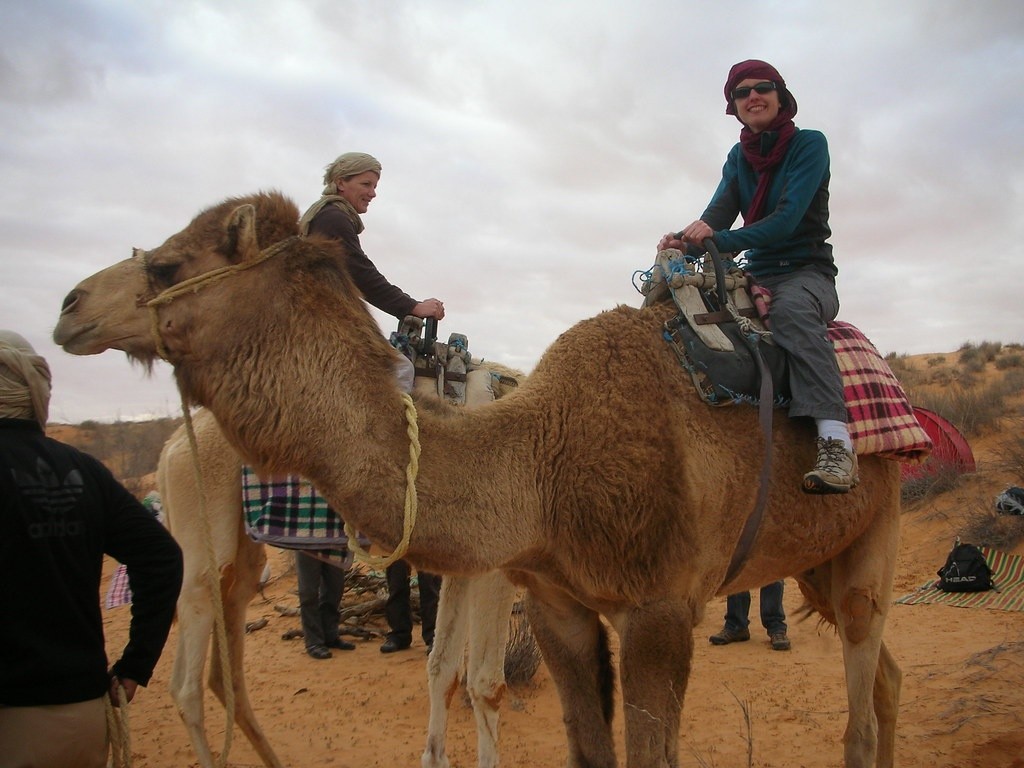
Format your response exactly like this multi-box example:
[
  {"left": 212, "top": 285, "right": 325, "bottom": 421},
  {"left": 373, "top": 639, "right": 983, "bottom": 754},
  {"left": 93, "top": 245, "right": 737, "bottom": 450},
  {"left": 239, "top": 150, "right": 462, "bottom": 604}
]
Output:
[
  {"left": 158, "top": 338, "right": 526, "bottom": 767},
  {"left": 51, "top": 188, "right": 902, "bottom": 768}
]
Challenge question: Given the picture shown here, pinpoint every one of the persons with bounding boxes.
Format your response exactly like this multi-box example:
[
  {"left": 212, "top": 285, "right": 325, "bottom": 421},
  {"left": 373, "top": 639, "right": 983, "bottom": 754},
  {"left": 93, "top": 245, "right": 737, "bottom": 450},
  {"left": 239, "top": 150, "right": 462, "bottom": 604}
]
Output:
[
  {"left": 293, "top": 548, "right": 358, "bottom": 660},
  {"left": 655, "top": 58, "right": 863, "bottom": 493},
  {"left": 0, "top": 328, "right": 187, "bottom": 768},
  {"left": 294, "top": 150, "right": 446, "bottom": 321},
  {"left": 379, "top": 554, "right": 445, "bottom": 658},
  {"left": 709, "top": 577, "right": 792, "bottom": 651}
]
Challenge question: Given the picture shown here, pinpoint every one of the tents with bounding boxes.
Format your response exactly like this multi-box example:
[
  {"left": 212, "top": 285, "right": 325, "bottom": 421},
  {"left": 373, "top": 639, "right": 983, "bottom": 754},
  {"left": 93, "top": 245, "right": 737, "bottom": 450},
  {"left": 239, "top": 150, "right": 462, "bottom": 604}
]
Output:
[{"left": 899, "top": 401, "right": 979, "bottom": 495}]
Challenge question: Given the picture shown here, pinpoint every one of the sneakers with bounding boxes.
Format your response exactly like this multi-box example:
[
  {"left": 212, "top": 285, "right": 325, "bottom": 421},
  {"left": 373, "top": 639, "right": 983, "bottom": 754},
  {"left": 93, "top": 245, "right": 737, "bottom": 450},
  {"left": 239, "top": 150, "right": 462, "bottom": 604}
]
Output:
[
  {"left": 709, "top": 629, "right": 751, "bottom": 645},
  {"left": 771, "top": 633, "right": 790, "bottom": 651},
  {"left": 801, "top": 437, "right": 860, "bottom": 494}
]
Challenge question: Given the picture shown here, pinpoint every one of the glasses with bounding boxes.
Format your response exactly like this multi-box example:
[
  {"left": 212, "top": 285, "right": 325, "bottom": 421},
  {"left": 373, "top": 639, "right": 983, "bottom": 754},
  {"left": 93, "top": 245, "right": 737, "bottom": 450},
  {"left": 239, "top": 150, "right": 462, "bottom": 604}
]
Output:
[{"left": 732, "top": 82, "right": 779, "bottom": 98}]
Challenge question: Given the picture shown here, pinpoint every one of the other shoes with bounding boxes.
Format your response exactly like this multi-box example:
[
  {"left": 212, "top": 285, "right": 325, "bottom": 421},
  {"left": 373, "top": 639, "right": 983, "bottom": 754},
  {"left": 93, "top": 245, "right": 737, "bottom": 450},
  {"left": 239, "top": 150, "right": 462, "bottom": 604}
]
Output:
[
  {"left": 326, "top": 641, "right": 355, "bottom": 650},
  {"left": 308, "top": 645, "right": 331, "bottom": 659},
  {"left": 380, "top": 640, "right": 410, "bottom": 653}
]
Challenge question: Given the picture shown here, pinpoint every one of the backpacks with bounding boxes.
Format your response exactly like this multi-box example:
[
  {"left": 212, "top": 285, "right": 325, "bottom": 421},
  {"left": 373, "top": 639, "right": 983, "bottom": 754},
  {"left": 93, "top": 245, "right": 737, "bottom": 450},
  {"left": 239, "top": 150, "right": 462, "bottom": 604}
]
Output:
[{"left": 937, "top": 541, "right": 1001, "bottom": 593}]
